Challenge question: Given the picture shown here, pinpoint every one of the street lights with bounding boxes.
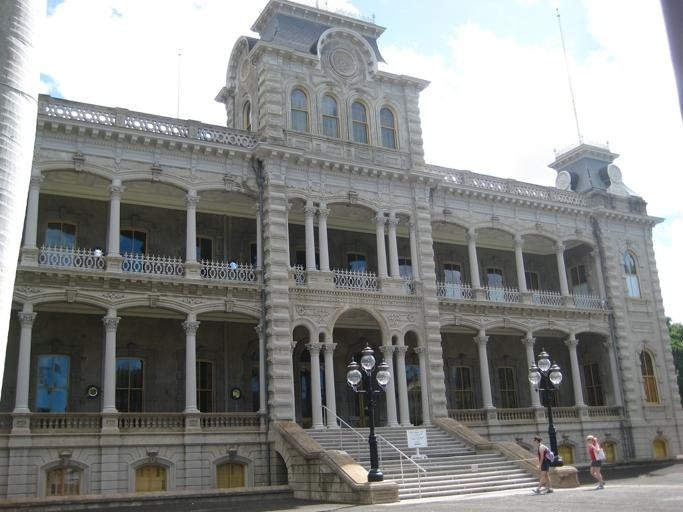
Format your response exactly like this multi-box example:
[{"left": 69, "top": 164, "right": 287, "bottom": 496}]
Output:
[
  {"left": 545, "top": 450, "right": 554, "bottom": 462},
  {"left": 593, "top": 448, "right": 606, "bottom": 461}
]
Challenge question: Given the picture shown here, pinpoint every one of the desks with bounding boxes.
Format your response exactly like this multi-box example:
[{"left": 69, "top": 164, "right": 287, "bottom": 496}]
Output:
[
  {"left": 344, "top": 340, "right": 391, "bottom": 483},
  {"left": 525, "top": 345, "right": 565, "bottom": 467}
]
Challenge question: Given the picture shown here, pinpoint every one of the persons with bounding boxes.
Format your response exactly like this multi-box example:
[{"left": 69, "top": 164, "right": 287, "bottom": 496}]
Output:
[
  {"left": 530, "top": 437, "right": 553, "bottom": 494},
  {"left": 584, "top": 434, "right": 605, "bottom": 490}
]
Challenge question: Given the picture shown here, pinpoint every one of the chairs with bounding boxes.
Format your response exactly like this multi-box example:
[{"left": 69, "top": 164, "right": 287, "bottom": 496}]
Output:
[
  {"left": 532, "top": 487, "right": 553, "bottom": 494},
  {"left": 597, "top": 481, "right": 605, "bottom": 490}
]
